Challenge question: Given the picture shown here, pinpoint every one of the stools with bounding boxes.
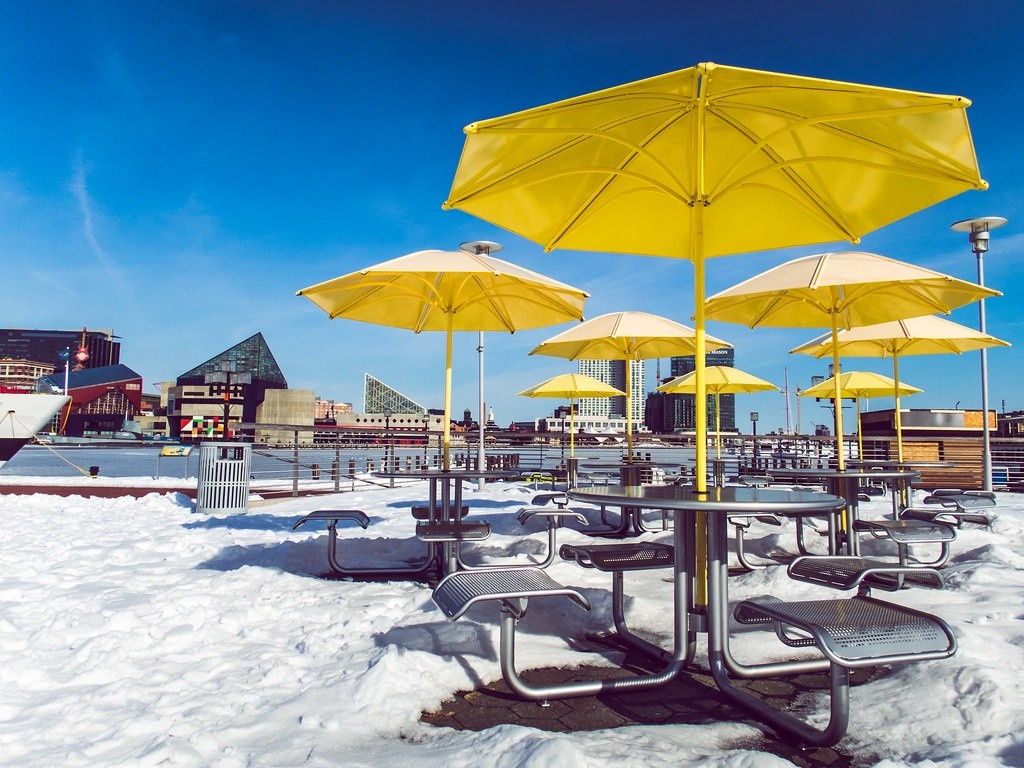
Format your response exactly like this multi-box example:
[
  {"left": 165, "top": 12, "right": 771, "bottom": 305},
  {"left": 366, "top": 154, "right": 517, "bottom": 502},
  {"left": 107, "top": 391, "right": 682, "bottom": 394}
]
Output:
[{"left": 292, "top": 476, "right": 997, "bottom": 748}]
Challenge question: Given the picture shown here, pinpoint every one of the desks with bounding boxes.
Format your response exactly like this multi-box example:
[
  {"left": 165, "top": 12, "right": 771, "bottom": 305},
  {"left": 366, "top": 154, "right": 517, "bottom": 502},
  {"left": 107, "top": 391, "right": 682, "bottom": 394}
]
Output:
[{"left": 370, "top": 460, "right": 959, "bottom": 676}]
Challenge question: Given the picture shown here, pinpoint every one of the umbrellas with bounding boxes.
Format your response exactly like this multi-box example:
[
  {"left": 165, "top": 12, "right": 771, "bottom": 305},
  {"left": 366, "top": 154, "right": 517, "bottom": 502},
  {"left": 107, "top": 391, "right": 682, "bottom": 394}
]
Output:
[
  {"left": 517, "top": 373, "right": 628, "bottom": 478},
  {"left": 789, "top": 311, "right": 1012, "bottom": 505},
  {"left": 796, "top": 371, "right": 924, "bottom": 485},
  {"left": 690, "top": 251, "right": 1002, "bottom": 530},
  {"left": 528, "top": 311, "right": 733, "bottom": 496},
  {"left": 442, "top": 63, "right": 990, "bottom": 615},
  {"left": 295, "top": 249, "right": 591, "bottom": 468},
  {"left": 655, "top": 366, "right": 781, "bottom": 459}
]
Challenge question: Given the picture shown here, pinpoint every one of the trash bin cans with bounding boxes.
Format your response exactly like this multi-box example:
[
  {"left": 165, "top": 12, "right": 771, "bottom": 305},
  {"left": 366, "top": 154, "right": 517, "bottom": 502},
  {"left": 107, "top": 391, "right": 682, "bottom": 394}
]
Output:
[{"left": 193, "top": 441, "right": 251, "bottom": 514}]
